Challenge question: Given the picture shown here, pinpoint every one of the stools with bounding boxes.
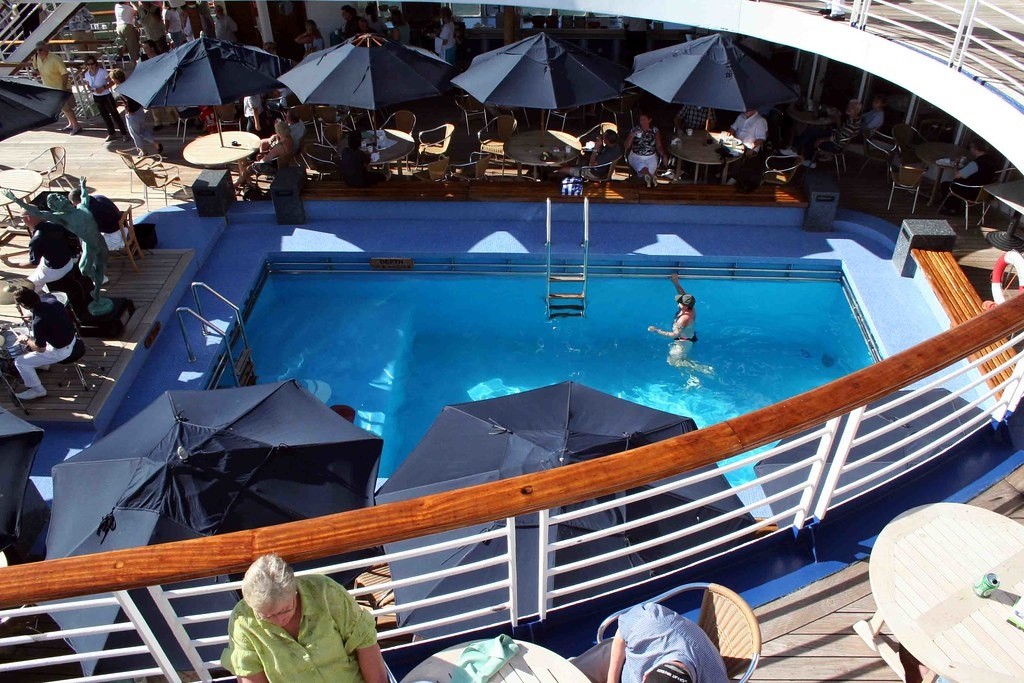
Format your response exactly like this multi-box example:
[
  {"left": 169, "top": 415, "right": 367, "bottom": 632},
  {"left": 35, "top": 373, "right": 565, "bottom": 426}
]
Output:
[{"left": 57, "top": 338, "right": 105, "bottom": 392}]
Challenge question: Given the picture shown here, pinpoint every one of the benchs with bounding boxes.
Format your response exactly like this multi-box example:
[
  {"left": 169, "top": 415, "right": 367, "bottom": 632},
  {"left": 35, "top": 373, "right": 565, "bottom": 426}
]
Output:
[{"left": 909, "top": 249, "right": 1018, "bottom": 404}]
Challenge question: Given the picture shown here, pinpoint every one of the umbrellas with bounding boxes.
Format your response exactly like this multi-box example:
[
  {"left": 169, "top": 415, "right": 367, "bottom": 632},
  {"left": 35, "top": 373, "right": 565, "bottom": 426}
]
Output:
[
  {"left": 752, "top": 386, "right": 992, "bottom": 530},
  {"left": 450, "top": 30, "right": 631, "bottom": 147},
  {"left": 112, "top": 31, "right": 287, "bottom": 149},
  {"left": 31, "top": 377, "right": 385, "bottom": 672},
  {"left": 374, "top": 381, "right": 758, "bottom": 643},
  {"left": 624, "top": 31, "right": 801, "bottom": 140},
  {"left": 274, "top": 31, "right": 460, "bottom": 150}
]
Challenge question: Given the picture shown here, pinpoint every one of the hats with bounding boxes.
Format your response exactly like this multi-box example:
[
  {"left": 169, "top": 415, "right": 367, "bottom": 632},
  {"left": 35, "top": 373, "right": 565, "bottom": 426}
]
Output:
[
  {"left": 644, "top": 662, "right": 692, "bottom": 683},
  {"left": 675, "top": 294, "right": 695, "bottom": 309}
]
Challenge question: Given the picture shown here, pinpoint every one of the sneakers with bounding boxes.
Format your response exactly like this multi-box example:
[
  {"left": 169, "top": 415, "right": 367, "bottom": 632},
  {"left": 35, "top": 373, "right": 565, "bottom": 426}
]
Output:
[
  {"left": 780, "top": 147, "right": 797, "bottom": 158},
  {"left": 804, "top": 159, "right": 816, "bottom": 168}
]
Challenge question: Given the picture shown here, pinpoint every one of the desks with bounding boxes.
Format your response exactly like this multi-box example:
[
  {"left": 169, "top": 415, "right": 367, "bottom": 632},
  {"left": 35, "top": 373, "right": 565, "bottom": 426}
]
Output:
[
  {"left": 853, "top": 501, "right": 1024, "bottom": 683},
  {"left": 914, "top": 142, "right": 970, "bottom": 207},
  {"left": 503, "top": 129, "right": 582, "bottom": 183},
  {"left": 0, "top": 169, "right": 43, "bottom": 228},
  {"left": 787, "top": 102, "right": 841, "bottom": 136},
  {"left": 396, "top": 638, "right": 593, "bottom": 683},
  {"left": 332, "top": 129, "right": 414, "bottom": 175},
  {"left": 667, "top": 129, "right": 744, "bottom": 185},
  {"left": 183, "top": 131, "right": 261, "bottom": 189}
]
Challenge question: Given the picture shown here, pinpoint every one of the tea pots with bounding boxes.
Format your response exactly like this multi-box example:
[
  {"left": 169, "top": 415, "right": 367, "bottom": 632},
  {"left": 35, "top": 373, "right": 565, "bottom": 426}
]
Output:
[{"left": 371, "top": 152, "right": 380, "bottom": 162}]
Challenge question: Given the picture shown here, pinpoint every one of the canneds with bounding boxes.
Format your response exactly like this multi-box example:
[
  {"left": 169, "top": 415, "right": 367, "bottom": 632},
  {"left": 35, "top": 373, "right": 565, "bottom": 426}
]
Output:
[{"left": 973, "top": 572, "right": 1000, "bottom": 597}]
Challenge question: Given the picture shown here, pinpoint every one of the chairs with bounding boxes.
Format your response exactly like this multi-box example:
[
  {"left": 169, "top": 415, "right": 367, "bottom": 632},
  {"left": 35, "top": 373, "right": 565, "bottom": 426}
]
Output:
[
  {"left": 109, "top": 86, "right": 1016, "bottom": 269},
  {"left": 597, "top": 582, "right": 762, "bottom": 683},
  {"left": 24, "top": 146, "right": 75, "bottom": 191}
]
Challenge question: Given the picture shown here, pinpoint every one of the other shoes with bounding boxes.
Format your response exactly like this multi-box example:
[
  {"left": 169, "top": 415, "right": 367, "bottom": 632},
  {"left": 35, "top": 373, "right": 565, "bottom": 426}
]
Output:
[
  {"left": 70, "top": 126, "right": 82, "bottom": 135},
  {"left": 62, "top": 125, "right": 72, "bottom": 130},
  {"left": 138, "top": 152, "right": 148, "bottom": 157},
  {"left": 645, "top": 174, "right": 651, "bottom": 187},
  {"left": 17, "top": 388, "right": 47, "bottom": 399},
  {"left": 122, "top": 133, "right": 129, "bottom": 143},
  {"left": 385, "top": 170, "right": 393, "bottom": 180},
  {"left": 106, "top": 133, "right": 115, "bottom": 140},
  {"left": 157, "top": 143, "right": 163, "bottom": 153},
  {"left": 651, "top": 174, "right": 659, "bottom": 186}
]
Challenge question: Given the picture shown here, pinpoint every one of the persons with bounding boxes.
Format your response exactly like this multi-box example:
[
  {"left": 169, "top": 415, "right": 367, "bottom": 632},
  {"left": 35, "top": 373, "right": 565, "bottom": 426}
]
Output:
[
  {"left": 0, "top": 0, "right": 103, "bottom": 68},
  {"left": 0, "top": 189, "right": 128, "bottom": 401},
  {"left": 670, "top": 273, "right": 695, "bottom": 326},
  {"left": 716, "top": 70, "right": 886, "bottom": 186},
  {"left": 213, "top": 555, "right": 390, "bottom": 683},
  {"left": 937, "top": 137, "right": 996, "bottom": 217},
  {"left": 31, "top": 41, "right": 164, "bottom": 158},
  {"left": 340, "top": 130, "right": 392, "bottom": 190},
  {"left": 557, "top": 105, "right": 716, "bottom": 187},
  {"left": 113, "top": 0, "right": 305, "bottom": 190},
  {"left": 294, "top": 0, "right": 454, "bottom": 66},
  {"left": 569, "top": 602, "right": 731, "bottom": 683},
  {"left": 0, "top": 176, "right": 108, "bottom": 304}
]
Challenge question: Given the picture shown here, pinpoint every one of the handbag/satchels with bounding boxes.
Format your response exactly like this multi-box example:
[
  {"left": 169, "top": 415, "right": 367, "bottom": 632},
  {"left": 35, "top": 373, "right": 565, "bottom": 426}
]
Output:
[{"left": 562, "top": 176, "right": 583, "bottom": 195}]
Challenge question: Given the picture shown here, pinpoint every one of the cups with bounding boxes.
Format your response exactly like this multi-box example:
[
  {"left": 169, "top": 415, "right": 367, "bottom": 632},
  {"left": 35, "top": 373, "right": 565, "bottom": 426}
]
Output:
[
  {"left": 635, "top": 131, "right": 642, "bottom": 138},
  {"left": 566, "top": 146, "right": 571, "bottom": 153},
  {"left": 361, "top": 142, "right": 366, "bottom": 148},
  {"left": 367, "top": 146, "right": 372, "bottom": 153},
  {"left": 687, "top": 129, "right": 692, "bottom": 136}
]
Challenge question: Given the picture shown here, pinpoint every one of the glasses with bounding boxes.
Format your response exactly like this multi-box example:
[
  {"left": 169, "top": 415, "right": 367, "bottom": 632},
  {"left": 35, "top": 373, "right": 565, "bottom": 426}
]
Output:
[
  {"left": 257, "top": 595, "right": 296, "bottom": 620},
  {"left": 86, "top": 62, "right": 96, "bottom": 66},
  {"left": 36, "top": 47, "right": 44, "bottom": 51}
]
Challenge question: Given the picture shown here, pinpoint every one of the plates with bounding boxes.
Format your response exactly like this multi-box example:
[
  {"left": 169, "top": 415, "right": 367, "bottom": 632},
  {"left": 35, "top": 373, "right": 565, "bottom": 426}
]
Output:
[{"left": 723, "top": 138, "right": 742, "bottom": 147}]
